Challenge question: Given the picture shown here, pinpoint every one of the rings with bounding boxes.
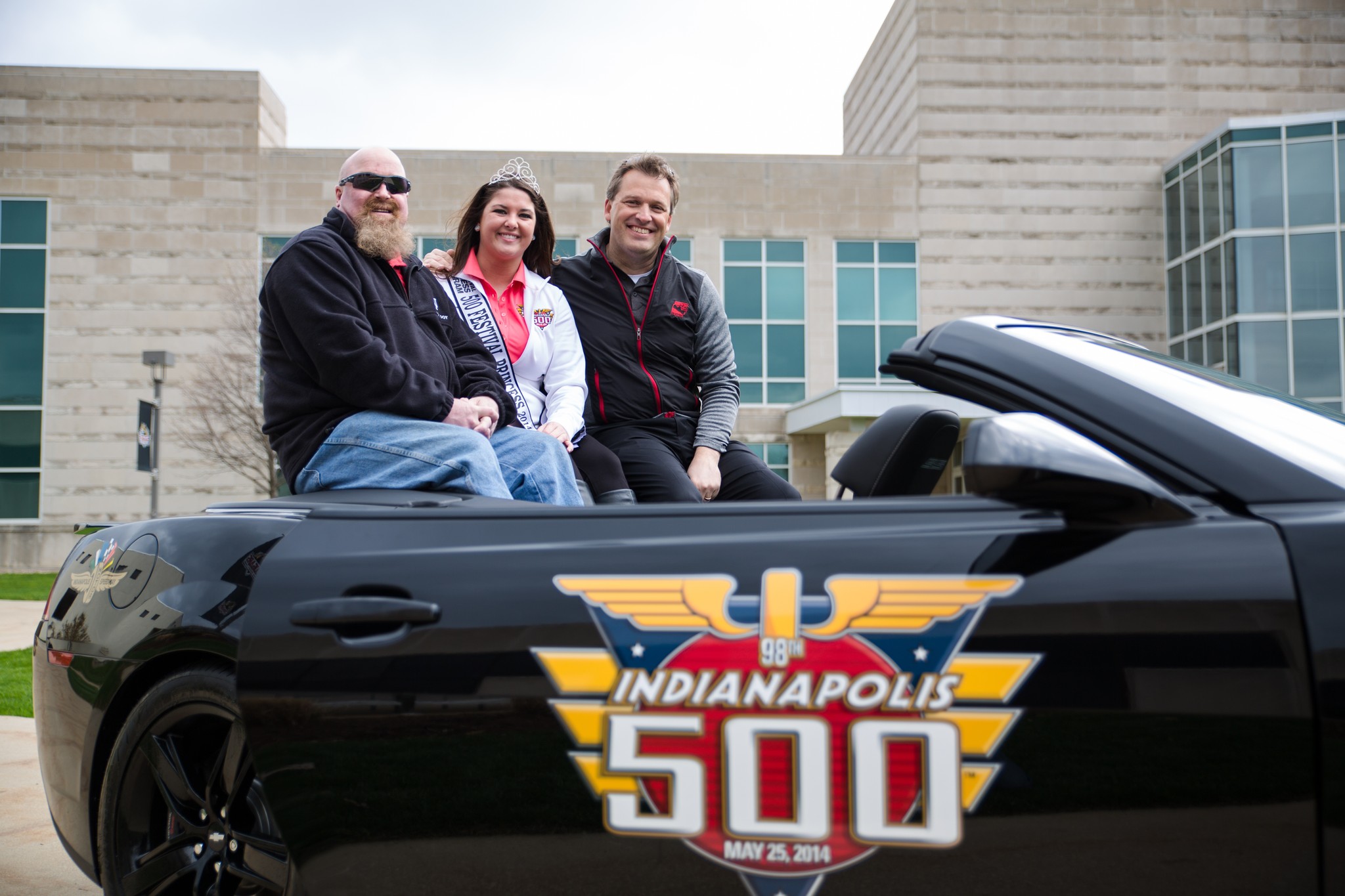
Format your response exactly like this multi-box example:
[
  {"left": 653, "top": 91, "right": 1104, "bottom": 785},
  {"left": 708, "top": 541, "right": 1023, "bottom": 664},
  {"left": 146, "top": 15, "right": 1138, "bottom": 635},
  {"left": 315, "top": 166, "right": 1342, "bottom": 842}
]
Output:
[{"left": 704, "top": 497, "right": 712, "bottom": 501}]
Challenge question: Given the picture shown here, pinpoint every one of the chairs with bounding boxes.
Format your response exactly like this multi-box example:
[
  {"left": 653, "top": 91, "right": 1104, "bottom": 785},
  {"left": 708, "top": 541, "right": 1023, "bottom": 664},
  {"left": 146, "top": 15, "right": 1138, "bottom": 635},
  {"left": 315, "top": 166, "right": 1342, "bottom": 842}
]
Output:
[{"left": 829, "top": 401, "right": 960, "bottom": 500}]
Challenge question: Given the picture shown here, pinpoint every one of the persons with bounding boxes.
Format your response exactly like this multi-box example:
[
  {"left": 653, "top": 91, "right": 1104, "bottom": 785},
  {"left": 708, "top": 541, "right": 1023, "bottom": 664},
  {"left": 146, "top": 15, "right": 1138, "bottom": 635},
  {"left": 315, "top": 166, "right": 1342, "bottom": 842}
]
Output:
[
  {"left": 422, "top": 153, "right": 804, "bottom": 507},
  {"left": 258, "top": 145, "right": 586, "bottom": 507},
  {"left": 421, "top": 157, "right": 637, "bottom": 507}
]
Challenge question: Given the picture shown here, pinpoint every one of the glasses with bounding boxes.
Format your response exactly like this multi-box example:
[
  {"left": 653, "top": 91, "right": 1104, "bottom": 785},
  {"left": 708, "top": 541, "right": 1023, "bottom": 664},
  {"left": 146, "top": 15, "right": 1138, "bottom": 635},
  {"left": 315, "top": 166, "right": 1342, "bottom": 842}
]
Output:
[{"left": 339, "top": 172, "right": 411, "bottom": 194}]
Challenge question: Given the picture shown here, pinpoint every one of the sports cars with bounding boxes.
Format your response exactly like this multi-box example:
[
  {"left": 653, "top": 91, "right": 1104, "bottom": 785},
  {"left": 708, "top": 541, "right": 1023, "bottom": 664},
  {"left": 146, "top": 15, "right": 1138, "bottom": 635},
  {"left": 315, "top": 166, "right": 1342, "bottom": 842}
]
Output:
[{"left": 34, "top": 312, "right": 1345, "bottom": 896}]
